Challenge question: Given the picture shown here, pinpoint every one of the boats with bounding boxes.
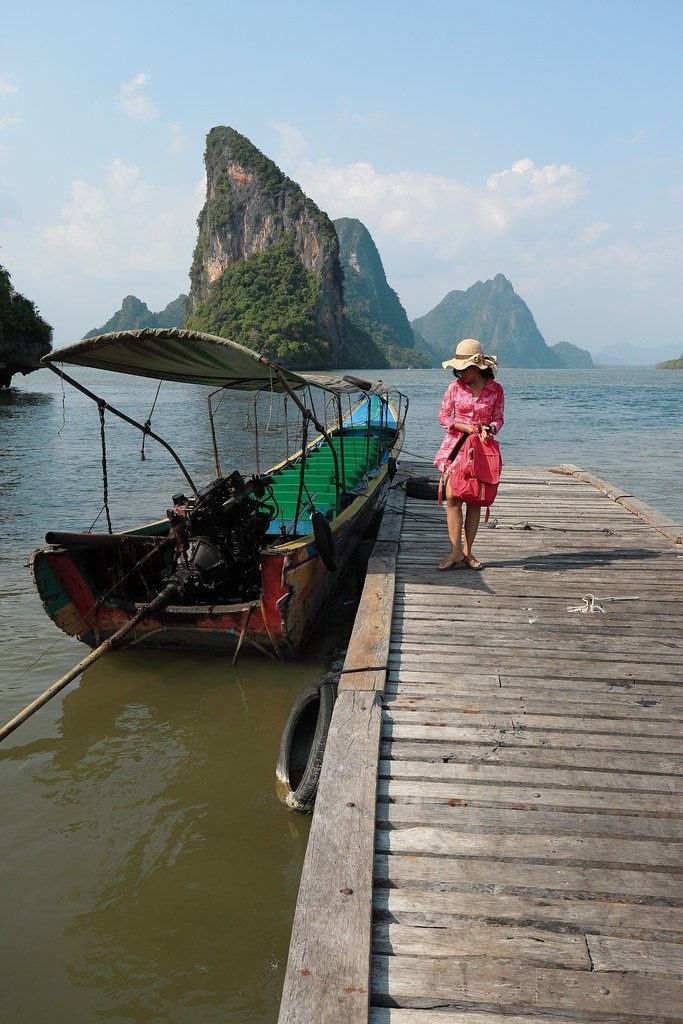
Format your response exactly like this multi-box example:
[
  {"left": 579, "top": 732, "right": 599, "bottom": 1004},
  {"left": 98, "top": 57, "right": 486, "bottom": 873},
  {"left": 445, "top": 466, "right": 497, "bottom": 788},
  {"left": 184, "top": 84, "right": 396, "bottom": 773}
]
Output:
[{"left": 33, "top": 324, "right": 410, "bottom": 658}]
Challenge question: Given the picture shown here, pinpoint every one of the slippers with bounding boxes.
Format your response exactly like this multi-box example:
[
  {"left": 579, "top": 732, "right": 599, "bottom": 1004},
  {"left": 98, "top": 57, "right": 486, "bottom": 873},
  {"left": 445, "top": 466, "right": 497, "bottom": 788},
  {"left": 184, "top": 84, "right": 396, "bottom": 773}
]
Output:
[
  {"left": 437, "top": 554, "right": 466, "bottom": 572},
  {"left": 466, "top": 555, "right": 483, "bottom": 570}
]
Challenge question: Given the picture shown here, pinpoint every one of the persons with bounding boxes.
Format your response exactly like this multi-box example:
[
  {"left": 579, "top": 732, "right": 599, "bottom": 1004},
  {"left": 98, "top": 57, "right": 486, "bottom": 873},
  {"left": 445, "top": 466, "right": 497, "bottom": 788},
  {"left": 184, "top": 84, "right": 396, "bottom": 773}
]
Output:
[{"left": 436, "top": 338, "right": 505, "bottom": 570}]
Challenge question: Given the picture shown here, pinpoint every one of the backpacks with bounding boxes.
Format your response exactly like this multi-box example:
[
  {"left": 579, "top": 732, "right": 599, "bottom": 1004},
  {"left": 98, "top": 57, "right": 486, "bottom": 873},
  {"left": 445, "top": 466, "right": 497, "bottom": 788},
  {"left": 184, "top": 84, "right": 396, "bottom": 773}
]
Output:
[{"left": 438, "top": 422, "right": 505, "bottom": 523}]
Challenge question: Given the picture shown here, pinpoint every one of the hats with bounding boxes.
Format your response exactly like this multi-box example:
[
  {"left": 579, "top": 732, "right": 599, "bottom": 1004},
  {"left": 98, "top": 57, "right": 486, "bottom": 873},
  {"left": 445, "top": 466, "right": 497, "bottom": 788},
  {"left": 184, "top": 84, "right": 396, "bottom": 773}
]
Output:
[{"left": 442, "top": 339, "right": 498, "bottom": 381}]
